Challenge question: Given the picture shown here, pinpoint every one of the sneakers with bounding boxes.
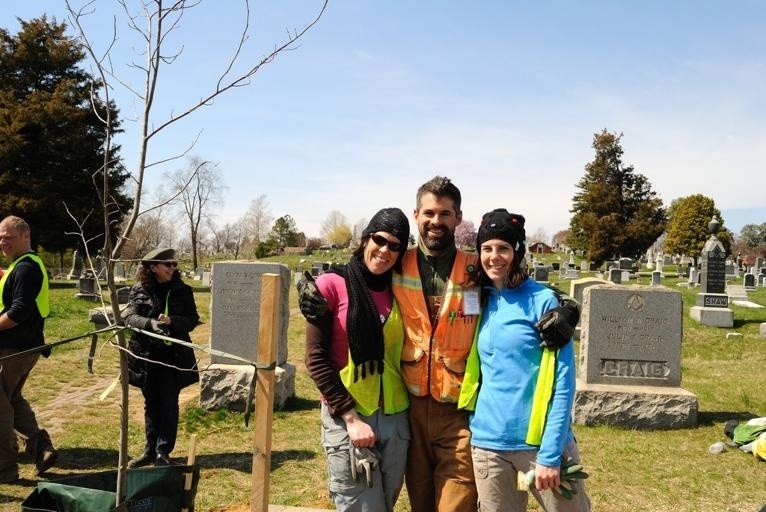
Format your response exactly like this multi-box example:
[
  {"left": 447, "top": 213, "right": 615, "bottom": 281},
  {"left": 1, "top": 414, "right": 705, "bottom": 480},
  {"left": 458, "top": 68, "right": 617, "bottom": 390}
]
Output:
[{"left": 127, "top": 452, "right": 170, "bottom": 467}]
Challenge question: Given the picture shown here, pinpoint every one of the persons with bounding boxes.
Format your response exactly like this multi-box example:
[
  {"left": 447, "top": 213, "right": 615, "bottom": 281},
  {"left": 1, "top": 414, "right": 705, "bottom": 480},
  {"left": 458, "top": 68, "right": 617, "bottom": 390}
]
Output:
[
  {"left": 302, "top": 207, "right": 412, "bottom": 512},
  {"left": 469, "top": 208, "right": 593, "bottom": 511},
  {"left": 296, "top": 176, "right": 581, "bottom": 511},
  {"left": 118, "top": 246, "right": 203, "bottom": 468},
  {"left": 0, "top": 215, "right": 58, "bottom": 484}
]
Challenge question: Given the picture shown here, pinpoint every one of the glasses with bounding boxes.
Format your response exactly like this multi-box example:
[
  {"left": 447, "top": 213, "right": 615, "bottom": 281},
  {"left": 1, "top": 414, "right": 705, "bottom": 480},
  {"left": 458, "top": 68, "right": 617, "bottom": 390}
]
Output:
[
  {"left": 157, "top": 261, "right": 177, "bottom": 267},
  {"left": 369, "top": 233, "right": 403, "bottom": 252}
]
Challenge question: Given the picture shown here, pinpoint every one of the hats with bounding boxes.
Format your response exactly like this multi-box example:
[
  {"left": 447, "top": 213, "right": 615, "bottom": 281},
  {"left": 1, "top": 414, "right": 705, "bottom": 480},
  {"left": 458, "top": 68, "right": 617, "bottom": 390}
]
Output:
[
  {"left": 141, "top": 247, "right": 176, "bottom": 265},
  {"left": 362, "top": 207, "right": 410, "bottom": 250},
  {"left": 475, "top": 208, "right": 526, "bottom": 254}
]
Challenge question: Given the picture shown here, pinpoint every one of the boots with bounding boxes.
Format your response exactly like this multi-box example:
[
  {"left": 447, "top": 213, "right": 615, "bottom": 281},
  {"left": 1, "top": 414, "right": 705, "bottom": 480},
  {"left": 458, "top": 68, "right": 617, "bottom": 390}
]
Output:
[{"left": 25, "top": 428, "right": 58, "bottom": 472}]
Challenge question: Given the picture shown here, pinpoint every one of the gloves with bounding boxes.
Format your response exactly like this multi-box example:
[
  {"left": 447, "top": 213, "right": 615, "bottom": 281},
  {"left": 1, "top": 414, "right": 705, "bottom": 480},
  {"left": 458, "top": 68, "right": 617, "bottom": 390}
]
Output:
[
  {"left": 349, "top": 437, "right": 383, "bottom": 489},
  {"left": 516, "top": 455, "right": 589, "bottom": 500},
  {"left": 534, "top": 299, "right": 579, "bottom": 351},
  {"left": 296, "top": 270, "right": 328, "bottom": 322}
]
